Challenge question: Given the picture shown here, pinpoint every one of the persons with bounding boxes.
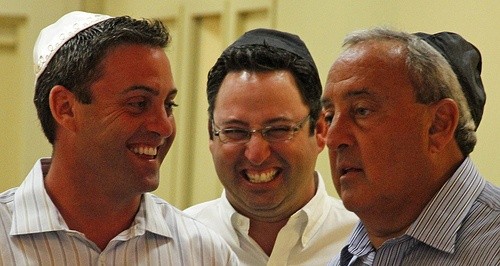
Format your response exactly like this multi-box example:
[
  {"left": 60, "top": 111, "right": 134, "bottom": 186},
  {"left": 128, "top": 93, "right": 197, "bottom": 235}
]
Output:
[
  {"left": 0, "top": 11, "right": 240, "bottom": 266},
  {"left": 321, "top": 26, "right": 500, "bottom": 266},
  {"left": 183, "top": 27, "right": 361, "bottom": 266}
]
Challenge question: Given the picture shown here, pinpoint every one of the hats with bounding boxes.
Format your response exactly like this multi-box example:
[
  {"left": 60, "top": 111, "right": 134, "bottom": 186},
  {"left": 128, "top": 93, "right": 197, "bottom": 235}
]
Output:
[
  {"left": 33, "top": 11, "right": 116, "bottom": 78},
  {"left": 221, "top": 29, "right": 323, "bottom": 98},
  {"left": 415, "top": 31, "right": 486, "bottom": 131}
]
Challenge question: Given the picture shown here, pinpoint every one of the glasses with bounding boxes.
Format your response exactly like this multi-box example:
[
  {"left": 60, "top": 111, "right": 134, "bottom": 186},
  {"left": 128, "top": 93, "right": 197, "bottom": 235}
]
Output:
[{"left": 210, "top": 110, "right": 313, "bottom": 146}]
